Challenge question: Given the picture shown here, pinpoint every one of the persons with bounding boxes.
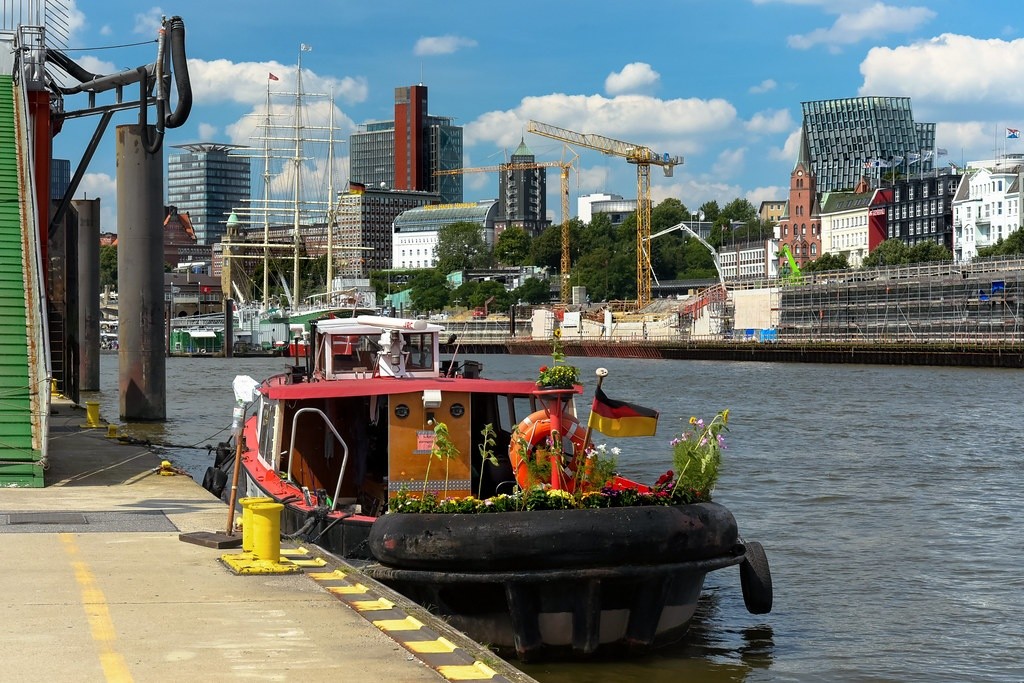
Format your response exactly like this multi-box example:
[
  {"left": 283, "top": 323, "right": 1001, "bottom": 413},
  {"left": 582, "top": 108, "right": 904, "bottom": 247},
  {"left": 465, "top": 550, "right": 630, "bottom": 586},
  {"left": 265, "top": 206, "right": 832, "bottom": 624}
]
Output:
[{"left": 585, "top": 294, "right": 592, "bottom": 309}]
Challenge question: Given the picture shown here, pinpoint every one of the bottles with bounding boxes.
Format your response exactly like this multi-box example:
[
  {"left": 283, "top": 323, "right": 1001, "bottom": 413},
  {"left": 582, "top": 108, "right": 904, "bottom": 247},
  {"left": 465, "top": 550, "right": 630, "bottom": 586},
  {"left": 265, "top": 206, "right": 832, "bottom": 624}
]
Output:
[{"left": 230, "top": 398, "right": 245, "bottom": 436}]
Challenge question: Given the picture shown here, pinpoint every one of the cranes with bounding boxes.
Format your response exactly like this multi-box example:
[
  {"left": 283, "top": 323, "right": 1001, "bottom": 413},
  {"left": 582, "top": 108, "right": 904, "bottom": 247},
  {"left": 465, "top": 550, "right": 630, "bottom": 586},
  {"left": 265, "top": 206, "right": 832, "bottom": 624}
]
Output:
[
  {"left": 431, "top": 140, "right": 587, "bottom": 311},
  {"left": 528, "top": 116, "right": 685, "bottom": 306}
]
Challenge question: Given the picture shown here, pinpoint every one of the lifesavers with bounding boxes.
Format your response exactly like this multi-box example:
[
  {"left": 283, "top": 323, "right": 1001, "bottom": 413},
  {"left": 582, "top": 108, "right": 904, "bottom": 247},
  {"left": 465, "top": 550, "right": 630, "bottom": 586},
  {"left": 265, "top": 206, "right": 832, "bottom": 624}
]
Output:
[
  {"left": 511, "top": 418, "right": 592, "bottom": 495},
  {"left": 508, "top": 408, "right": 586, "bottom": 459},
  {"left": 201, "top": 348, "right": 206, "bottom": 354}
]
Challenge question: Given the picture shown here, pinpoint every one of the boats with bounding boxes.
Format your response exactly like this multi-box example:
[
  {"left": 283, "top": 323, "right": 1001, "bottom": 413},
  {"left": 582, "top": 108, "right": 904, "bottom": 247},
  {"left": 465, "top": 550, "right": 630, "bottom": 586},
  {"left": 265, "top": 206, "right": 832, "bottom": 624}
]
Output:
[{"left": 232, "top": 288, "right": 777, "bottom": 652}]
